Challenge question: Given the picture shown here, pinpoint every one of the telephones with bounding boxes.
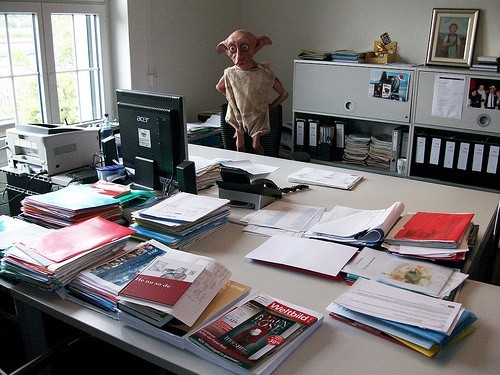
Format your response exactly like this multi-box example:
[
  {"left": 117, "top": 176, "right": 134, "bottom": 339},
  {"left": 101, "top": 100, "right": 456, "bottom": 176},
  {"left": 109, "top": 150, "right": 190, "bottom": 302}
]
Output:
[{"left": 216, "top": 168, "right": 282, "bottom": 212}]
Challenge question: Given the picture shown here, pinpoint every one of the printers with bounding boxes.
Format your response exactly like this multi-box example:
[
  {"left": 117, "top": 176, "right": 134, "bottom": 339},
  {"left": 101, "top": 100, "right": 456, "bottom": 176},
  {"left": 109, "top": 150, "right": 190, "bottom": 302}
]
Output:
[{"left": 4, "top": 123, "right": 100, "bottom": 177}]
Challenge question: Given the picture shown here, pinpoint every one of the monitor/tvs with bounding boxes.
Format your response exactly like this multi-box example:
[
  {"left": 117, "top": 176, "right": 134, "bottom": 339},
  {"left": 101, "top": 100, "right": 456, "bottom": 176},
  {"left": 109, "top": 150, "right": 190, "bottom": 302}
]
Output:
[{"left": 115, "top": 88, "right": 198, "bottom": 193}]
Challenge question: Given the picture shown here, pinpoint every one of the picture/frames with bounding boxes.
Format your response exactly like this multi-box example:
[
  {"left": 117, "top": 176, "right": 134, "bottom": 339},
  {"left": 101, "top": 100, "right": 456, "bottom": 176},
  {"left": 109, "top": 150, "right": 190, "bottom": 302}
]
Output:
[{"left": 426, "top": 7, "right": 480, "bottom": 69}]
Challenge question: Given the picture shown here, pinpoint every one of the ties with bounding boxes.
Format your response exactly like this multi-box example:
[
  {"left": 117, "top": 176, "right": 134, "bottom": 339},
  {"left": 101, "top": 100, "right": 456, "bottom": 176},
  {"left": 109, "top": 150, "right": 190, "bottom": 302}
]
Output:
[{"left": 491, "top": 93, "right": 492, "bottom": 108}]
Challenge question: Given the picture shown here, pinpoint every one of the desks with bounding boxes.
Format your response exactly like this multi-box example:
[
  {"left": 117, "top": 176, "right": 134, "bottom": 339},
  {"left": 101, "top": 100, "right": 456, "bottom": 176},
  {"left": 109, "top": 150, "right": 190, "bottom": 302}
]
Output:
[{"left": 0, "top": 129, "right": 500, "bottom": 375}]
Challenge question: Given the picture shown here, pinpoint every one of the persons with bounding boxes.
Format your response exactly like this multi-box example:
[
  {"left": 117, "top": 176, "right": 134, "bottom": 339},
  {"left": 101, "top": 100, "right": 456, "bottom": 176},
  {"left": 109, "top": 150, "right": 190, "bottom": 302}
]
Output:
[
  {"left": 495, "top": 89, "right": 500, "bottom": 110},
  {"left": 214, "top": 28, "right": 291, "bottom": 157},
  {"left": 484, "top": 85, "right": 497, "bottom": 110},
  {"left": 471, "top": 84, "right": 486, "bottom": 109},
  {"left": 441, "top": 23, "right": 463, "bottom": 59}
]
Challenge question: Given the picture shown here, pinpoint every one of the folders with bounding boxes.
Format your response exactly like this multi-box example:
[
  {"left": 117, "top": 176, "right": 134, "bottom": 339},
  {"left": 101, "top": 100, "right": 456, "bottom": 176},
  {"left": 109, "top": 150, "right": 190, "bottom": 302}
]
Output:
[
  {"left": 415, "top": 132, "right": 500, "bottom": 187},
  {"left": 390, "top": 125, "right": 408, "bottom": 172},
  {"left": 296, "top": 113, "right": 318, "bottom": 159},
  {"left": 334, "top": 120, "right": 350, "bottom": 160}
]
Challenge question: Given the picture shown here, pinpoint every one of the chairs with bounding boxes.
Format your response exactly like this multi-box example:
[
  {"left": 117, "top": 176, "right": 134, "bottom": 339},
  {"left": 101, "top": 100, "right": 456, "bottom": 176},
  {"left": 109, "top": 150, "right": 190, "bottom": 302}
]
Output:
[{"left": 220, "top": 102, "right": 311, "bottom": 162}]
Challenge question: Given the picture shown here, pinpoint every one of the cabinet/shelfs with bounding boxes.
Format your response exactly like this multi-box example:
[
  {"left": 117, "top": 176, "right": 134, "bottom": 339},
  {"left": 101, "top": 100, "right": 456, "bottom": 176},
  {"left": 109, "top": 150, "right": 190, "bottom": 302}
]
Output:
[{"left": 290, "top": 59, "right": 500, "bottom": 192}]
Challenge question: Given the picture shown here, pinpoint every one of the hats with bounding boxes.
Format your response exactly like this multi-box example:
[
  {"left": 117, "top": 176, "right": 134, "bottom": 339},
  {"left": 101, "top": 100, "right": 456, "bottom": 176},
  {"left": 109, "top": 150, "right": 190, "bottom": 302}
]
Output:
[{"left": 489, "top": 86, "right": 496, "bottom": 90}]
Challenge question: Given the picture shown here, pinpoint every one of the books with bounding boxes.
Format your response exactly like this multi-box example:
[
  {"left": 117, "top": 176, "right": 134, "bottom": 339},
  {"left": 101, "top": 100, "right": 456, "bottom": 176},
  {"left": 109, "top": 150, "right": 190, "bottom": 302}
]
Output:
[
  {"left": 0, "top": 175, "right": 500, "bottom": 375},
  {"left": 469, "top": 56, "right": 498, "bottom": 72},
  {"left": 298, "top": 48, "right": 329, "bottom": 61},
  {"left": 330, "top": 49, "right": 365, "bottom": 63},
  {"left": 287, "top": 166, "right": 364, "bottom": 191},
  {"left": 186, "top": 124, "right": 210, "bottom": 143}
]
